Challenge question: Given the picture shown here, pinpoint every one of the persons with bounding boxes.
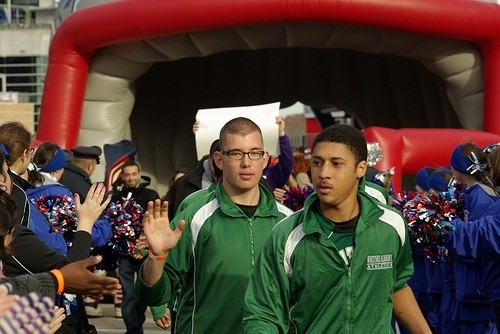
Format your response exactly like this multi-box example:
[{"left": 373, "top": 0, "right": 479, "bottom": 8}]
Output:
[
  {"left": 242, "top": 124, "right": 436, "bottom": 334},
  {"left": 105, "top": 162, "right": 162, "bottom": 334},
  {"left": 132, "top": 117, "right": 301, "bottom": 334},
  {"left": 0, "top": 116, "right": 500, "bottom": 334}
]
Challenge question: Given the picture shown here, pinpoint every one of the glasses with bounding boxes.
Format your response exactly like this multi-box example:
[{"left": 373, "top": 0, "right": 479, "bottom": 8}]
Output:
[
  {"left": 222, "top": 149, "right": 265, "bottom": 161},
  {"left": 27, "top": 148, "right": 36, "bottom": 153}
]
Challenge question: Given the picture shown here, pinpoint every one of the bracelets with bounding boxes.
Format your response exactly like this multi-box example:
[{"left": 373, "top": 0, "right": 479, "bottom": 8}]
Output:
[
  {"left": 147, "top": 248, "right": 170, "bottom": 260},
  {"left": 50, "top": 270, "right": 64, "bottom": 294}
]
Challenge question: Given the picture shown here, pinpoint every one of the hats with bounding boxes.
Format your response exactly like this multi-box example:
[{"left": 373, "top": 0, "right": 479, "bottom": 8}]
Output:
[{"left": 70, "top": 145, "right": 102, "bottom": 164}]
